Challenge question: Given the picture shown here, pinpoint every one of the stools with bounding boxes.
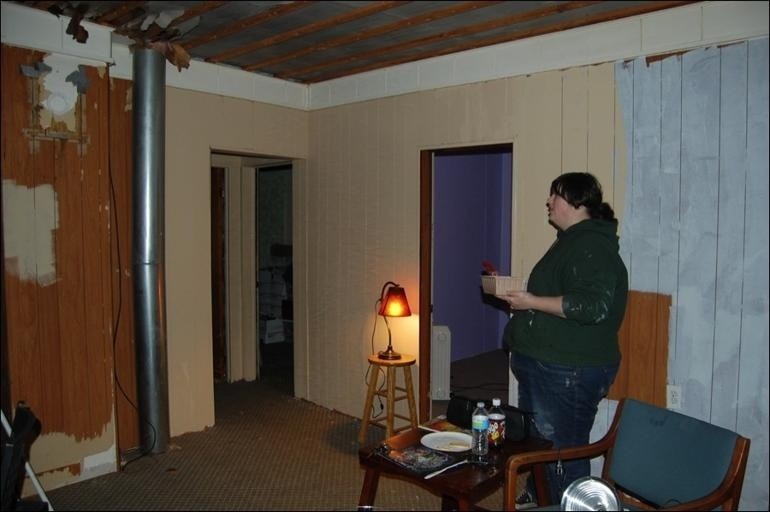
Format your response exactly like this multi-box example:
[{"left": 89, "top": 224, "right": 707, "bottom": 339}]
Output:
[{"left": 357, "top": 354, "right": 419, "bottom": 444}]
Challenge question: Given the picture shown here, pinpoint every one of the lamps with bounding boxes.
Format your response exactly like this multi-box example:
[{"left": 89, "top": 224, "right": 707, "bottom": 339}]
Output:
[{"left": 377, "top": 280, "right": 411, "bottom": 360}]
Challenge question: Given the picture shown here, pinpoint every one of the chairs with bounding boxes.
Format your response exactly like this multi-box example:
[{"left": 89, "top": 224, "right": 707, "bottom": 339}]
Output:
[{"left": 503, "top": 395, "right": 750, "bottom": 512}]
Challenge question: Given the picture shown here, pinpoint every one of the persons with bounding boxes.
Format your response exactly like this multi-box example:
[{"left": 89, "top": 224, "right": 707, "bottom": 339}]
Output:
[{"left": 495, "top": 170, "right": 630, "bottom": 510}]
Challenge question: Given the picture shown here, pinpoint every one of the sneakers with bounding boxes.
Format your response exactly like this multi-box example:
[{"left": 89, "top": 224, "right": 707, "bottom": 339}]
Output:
[{"left": 514, "top": 490, "right": 536, "bottom": 508}]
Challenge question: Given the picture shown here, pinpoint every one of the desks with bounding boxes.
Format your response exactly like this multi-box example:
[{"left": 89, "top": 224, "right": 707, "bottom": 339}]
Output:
[{"left": 356, "top": 414, "right": 554, "bottom": 511}]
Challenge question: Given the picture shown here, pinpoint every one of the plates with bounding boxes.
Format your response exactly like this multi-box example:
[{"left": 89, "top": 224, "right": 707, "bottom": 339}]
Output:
[{"left": 421, "top": 431, "right": 477, "bottom": 453}]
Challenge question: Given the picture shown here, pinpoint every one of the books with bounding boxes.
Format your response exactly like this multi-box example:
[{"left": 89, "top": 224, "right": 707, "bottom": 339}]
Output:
[
  {"left": 417, "top": 417, "right": 463, "bottom": 434},
  {"left": 377, "top": 443, "right": 458, "bottom": 475}
]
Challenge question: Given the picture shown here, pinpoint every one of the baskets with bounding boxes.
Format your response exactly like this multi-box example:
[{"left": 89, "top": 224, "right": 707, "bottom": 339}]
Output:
[{"left": 481, "top": 274, "right": 526, "bottom": 296}]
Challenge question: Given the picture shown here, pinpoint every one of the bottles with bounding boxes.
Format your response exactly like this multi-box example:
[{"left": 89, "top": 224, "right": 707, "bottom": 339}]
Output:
[{"left": 472, "top": 398, "right": 505, "bottom": 456}]
